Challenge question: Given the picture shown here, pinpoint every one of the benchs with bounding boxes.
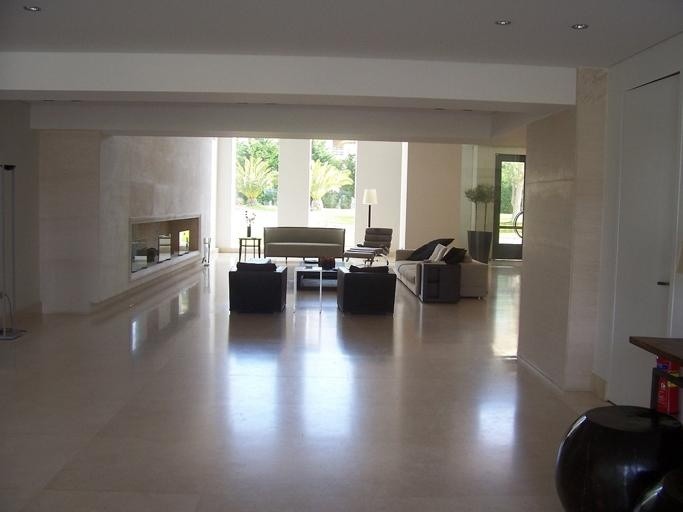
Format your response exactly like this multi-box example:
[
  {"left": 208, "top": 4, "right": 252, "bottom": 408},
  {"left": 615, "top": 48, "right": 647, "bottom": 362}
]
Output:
[{"left": 264, "top": 226, "right": 345, "bottom": 264}]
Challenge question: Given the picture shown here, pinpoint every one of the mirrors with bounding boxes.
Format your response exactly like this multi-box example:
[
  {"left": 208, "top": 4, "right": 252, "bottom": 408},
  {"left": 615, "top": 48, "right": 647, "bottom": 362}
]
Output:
[
  {"left": 157, "top": 232, "right": 172, "bottom": 263},
  {"left": 178, "top": 230, "right": 191, "bottom": 256},
  {"left": 130, "top": 241, "right": 148, "bottom": 272}
]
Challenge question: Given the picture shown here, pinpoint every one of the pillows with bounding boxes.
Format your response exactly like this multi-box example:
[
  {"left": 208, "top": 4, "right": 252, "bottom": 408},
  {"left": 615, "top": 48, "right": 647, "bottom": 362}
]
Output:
[
  {"left": 349, "top": 265, "right": 389, "bottom": 273},
  {"left": 443, "top": 246, "right": 466, "bottom": 263},
  {"left": 237, "top": 262, "right": 276, "bottom": 272},
  {"left": 408, "top": 238, "right": 455, "bottom": 261},
  {"left": 429, "top": 243, "right": 447, "bottom": 263}
]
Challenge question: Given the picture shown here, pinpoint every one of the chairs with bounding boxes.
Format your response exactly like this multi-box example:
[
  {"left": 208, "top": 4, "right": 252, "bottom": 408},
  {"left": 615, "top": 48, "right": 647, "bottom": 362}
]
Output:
[
  {"left": 229, "top": 266, "right": 288, "bottom": 313},
  {"left": 344, "top": 227, "right": 393, "bottom": 265},
  {"left": 336, "top": 266, "right": 397, "bottom": 315}
]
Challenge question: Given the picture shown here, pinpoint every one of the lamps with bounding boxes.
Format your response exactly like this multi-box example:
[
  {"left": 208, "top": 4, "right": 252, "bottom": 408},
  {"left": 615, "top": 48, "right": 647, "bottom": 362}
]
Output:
[{"left": 362, "top": 188, "right": 378, "bottom": 227}]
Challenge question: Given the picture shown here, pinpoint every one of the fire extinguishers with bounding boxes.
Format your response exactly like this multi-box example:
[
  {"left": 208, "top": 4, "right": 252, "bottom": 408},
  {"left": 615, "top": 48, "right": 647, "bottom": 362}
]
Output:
[{"left": 656, "top": 354, "right": 679, "bottom": 414}]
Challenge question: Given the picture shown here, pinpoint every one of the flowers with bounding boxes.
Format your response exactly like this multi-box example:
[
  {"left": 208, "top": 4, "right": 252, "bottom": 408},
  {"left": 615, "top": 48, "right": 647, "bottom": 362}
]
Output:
[{"left": 245, "top": 210, "right": 256, "bottom": 226}]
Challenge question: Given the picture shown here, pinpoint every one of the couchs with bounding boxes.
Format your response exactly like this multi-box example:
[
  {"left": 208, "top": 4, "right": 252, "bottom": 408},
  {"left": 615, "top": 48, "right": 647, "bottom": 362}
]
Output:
[{"left": 395, "top": 249, "right": 489, "bottom": 303}]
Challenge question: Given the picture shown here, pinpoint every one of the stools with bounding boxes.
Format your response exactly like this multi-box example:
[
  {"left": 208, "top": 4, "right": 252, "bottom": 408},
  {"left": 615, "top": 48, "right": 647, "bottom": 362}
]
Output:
[
  {"left": 624, "top": 466, "right": 683, "bottom": 511},
  {"left": 554, "top": 404, "right": 681, "bottom": 511}
]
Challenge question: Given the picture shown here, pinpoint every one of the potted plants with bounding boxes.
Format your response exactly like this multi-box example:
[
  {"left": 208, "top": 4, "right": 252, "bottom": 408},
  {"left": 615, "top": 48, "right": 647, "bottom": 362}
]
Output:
[{"left": 464, "top": 184, "right": 494, "bottom": 265}]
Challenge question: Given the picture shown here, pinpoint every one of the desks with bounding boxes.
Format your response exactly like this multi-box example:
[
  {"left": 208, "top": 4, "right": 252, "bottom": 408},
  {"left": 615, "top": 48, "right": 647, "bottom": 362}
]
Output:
[
  {"left": 629, "top": 335, "right": 683, "bottom": 409},
  {"left": 293, "top": 268, "right": 323, "bottom": 314},
  {"left": 238, "top": 237, "right": 261, "bottom": 261}
]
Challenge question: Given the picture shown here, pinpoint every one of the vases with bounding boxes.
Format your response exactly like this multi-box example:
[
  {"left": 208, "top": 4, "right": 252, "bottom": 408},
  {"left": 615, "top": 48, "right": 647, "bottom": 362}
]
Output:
[{"left": 247, "top": 226, "right": 252, "bottom": 237}]
